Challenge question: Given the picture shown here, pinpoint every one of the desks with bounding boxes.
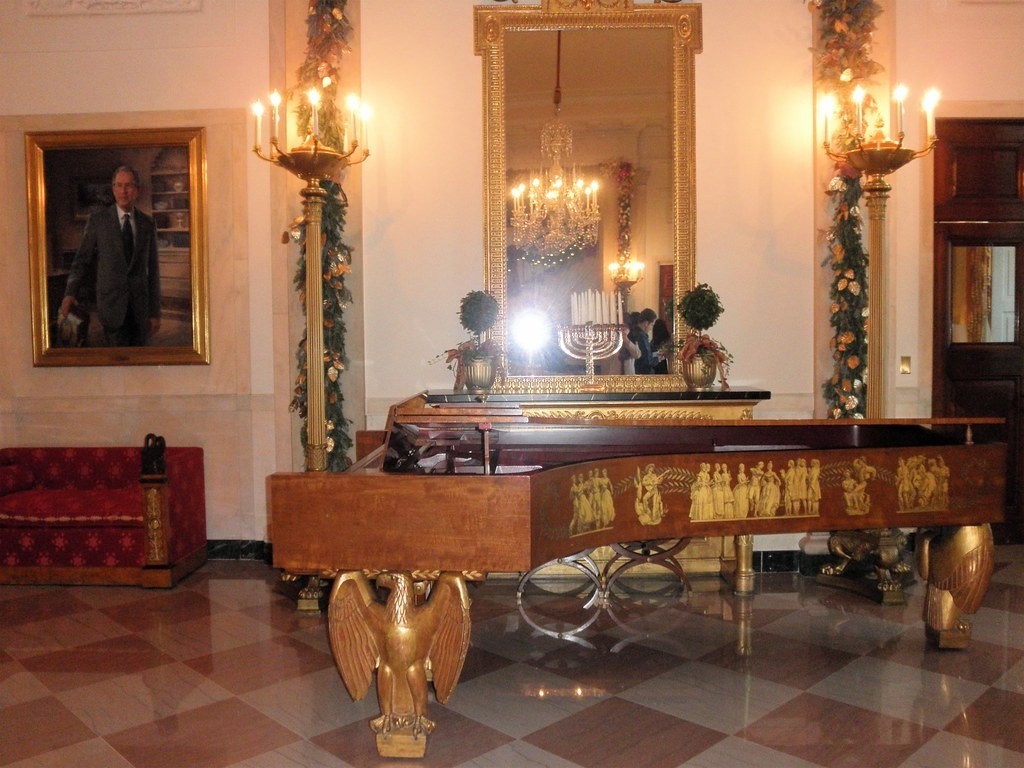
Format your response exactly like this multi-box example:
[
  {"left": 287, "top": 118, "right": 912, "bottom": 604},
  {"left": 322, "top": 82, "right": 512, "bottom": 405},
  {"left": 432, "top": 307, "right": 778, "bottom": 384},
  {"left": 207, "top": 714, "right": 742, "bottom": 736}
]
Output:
[{"left": 383, "top": 384, "right": 772, "bottom": 422}]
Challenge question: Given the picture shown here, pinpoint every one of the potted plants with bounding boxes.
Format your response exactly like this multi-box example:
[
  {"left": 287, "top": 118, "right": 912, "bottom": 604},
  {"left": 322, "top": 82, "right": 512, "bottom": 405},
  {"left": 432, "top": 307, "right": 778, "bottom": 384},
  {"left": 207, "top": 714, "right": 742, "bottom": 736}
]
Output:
[
  {"left": 677, "top": 281, "right": 736, "bottom": 392},
  {"left": 428, "top": 289, "right": 508, "bottom": 394}
]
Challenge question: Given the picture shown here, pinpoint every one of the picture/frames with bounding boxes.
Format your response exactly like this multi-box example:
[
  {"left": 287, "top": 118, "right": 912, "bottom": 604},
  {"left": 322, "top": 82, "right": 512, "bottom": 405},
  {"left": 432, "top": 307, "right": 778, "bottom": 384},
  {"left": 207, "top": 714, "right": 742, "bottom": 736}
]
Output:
[{"left": 24, "top": 125, "right": 212, "bottom": 368}]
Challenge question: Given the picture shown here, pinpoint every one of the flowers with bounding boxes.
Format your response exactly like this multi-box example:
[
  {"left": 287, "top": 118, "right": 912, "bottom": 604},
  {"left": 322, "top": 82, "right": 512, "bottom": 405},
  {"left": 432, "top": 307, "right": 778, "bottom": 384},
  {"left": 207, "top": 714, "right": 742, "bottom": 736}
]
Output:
[
  {"left": 614, "top": 160, "right": 637, "bottom": 263},
  {"left": 802, "top": 0, "right": 885, "bottom": 83},
  {"left": 819, "top": 162, "right": 868, "bottom": 420}
]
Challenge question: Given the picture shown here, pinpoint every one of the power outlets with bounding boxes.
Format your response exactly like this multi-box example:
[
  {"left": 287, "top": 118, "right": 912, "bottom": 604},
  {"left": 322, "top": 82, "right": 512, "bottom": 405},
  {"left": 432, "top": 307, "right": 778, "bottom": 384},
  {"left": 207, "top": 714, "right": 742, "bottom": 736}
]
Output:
[{"left": 900, "top": 356, "right": 912, "bottom": 374}]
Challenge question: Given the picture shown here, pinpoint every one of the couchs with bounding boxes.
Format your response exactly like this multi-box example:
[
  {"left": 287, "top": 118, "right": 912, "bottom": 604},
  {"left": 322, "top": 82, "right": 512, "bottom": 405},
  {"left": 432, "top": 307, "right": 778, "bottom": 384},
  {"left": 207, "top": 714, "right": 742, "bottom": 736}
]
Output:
[{"left": 0, "top": 445, "right": 208, "bottom": 589}]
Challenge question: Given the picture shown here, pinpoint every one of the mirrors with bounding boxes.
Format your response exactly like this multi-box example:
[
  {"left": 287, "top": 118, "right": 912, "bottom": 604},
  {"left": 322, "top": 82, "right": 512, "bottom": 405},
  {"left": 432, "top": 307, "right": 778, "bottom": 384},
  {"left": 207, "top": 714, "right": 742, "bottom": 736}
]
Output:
[{"left": 473, "top": 0, "right": 705, "bottom": 393}]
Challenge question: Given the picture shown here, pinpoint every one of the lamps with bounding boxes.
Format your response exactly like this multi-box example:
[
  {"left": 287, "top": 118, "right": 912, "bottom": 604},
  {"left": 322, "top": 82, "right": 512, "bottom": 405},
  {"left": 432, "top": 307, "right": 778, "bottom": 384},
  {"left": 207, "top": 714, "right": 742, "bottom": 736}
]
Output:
[
  {"left": 252, "top": 88, "right": 374, "bottom": 474},
  {"left": 609, "top": 262, "right": 646, "bottom": 324},
  {"left": 511, "top": 28, "right": 605, "bottom": 270},
  {"left": 819, "top": 81, "right": 944, "bottom": 421}
]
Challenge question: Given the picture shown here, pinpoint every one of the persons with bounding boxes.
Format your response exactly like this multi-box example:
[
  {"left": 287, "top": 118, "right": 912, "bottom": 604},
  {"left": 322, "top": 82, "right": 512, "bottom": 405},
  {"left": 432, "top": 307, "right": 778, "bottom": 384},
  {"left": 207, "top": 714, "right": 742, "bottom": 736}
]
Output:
[
  {"left": 650, "top": 319, "right": 671, "bottom": 374},
  {"left": 619, "top": 312, "right": 642, "bottom": 375},
  {"left": 628, "top": 310, "right": 642, "bottom": 332},
  {"left": 626, "top": 307, "right": 657, "bottom": 375},
  {"left": 60, "top": 165, "right": 161, "bottom": 347}
]
